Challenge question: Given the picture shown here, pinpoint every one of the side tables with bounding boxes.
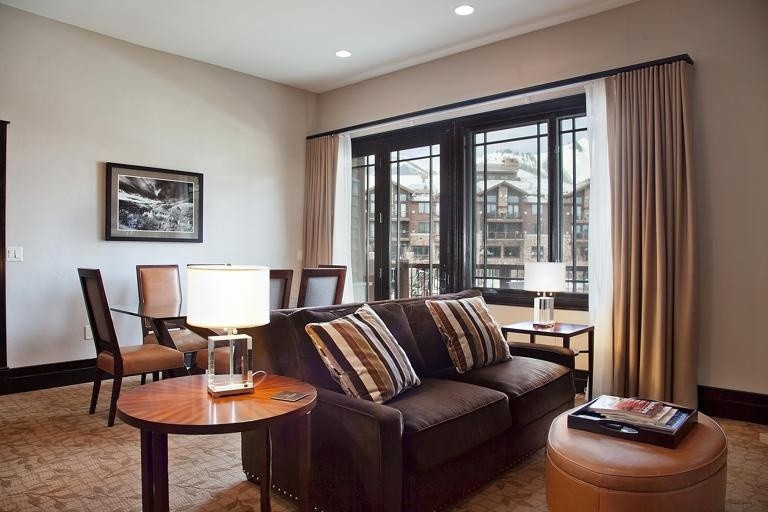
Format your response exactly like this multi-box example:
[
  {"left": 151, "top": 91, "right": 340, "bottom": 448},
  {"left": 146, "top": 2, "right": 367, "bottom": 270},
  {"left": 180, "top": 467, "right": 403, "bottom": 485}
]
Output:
[
  {"left": 501, "top": 321, "right": 594, "bottom": 402},
  {"left": 115, "top": 374, "right": 318, "bottom": 512}
]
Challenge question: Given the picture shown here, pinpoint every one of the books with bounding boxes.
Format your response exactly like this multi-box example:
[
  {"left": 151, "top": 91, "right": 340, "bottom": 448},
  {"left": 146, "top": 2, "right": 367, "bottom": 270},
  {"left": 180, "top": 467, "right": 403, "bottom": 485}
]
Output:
[{"left": 588, "top": 395, "right": 688, "bottom": 433}]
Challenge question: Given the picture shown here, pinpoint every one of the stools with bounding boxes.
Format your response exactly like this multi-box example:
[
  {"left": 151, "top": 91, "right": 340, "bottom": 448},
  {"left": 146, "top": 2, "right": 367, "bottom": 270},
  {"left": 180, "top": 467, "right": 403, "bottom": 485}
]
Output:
[{"left": 545, "top": 403, "right": 728, "bottom": 512}]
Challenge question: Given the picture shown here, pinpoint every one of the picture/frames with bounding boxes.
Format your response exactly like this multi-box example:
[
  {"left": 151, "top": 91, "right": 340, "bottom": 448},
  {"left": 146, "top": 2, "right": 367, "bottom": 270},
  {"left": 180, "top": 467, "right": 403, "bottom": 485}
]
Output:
[{"left": 106, "top": 162, "right": 203, "bottom": 243}]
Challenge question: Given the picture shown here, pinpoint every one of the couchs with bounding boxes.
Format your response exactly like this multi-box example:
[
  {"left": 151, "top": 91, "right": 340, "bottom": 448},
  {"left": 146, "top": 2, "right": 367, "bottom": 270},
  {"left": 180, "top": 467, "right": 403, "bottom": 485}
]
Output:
[{"left": 241, "top": 289, "right": 576, "bottom": 511}]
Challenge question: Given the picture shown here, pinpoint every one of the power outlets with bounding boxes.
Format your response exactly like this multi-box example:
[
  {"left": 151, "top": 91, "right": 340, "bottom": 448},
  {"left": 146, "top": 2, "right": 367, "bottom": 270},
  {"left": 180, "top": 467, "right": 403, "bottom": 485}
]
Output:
[{"left": 84, "top": 326, "right": 94, "bottom": 340}]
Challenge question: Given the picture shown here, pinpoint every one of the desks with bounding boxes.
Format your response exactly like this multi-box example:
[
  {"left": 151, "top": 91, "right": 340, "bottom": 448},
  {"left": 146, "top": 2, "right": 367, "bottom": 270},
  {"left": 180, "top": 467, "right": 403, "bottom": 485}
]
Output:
[{"left": 110, "top": 300, "right": 300, "bottom": 378}]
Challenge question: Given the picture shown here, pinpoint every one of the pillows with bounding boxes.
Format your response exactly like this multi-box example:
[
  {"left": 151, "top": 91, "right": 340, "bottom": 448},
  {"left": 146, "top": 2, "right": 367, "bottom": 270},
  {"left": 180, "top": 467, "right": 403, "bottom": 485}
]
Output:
[
  {"left": 305, "top": 303, "right": 421, "bottom": 405},
  {"left": 425, "top": 295, "right": 513, "bottom": 374},
  {"left": 289, "top": 302, "right": 424, "bottom": 389},
  {"left": 403, "top": 288, "right": 482, "bottom": 370}
]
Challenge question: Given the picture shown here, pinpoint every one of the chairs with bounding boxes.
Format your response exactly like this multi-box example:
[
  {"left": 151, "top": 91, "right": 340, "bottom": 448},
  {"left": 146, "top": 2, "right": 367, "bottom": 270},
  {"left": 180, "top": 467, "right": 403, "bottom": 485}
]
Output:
[
  {"left": 318, "top": 265, "right": 347, "bottom": 268},
  {"left": 196, "top": 270, "right": 293, "bottom": 375},
  {"left": 77, "top": 268, "right": 184, "bottom": 427},
  {"left": 297, "top": 268, "right": 346, "bottom": 308},
  {"left": 136, "top": 265, "right": 208, "bottom": 385}
]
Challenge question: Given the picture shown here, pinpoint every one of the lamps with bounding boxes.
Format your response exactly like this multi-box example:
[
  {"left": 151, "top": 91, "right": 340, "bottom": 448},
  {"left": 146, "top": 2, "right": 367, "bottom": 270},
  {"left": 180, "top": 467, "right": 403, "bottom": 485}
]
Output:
[
  {"left": 524, "top": 262, "right": 566, "bottom": 328},
  {"left": 187, "top": 263, "right": 271, "bottom": 398}
]
